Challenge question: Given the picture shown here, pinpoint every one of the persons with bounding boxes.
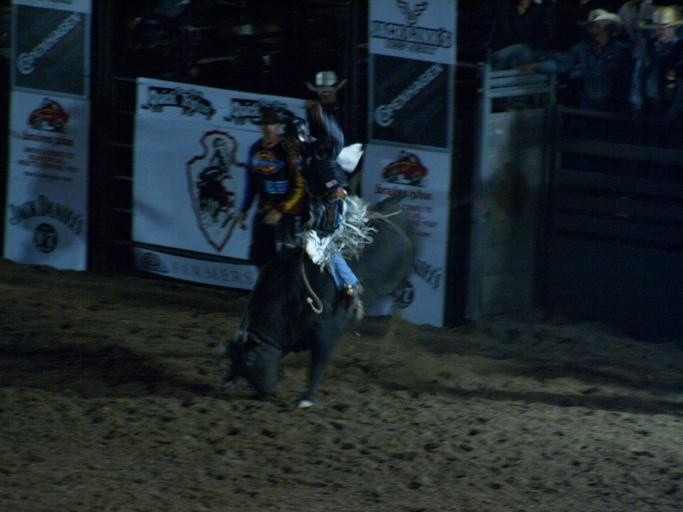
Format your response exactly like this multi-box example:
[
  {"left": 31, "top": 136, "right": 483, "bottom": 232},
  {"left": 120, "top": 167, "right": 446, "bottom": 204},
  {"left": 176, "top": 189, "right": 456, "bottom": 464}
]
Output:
[
  {"left": 235, "top": 107, "right": 309, "bottom": 268},
  {"left": 281, "top": 102, "right": 367, "bottom": 300},
  {"left": 87, "top": 1, "right": 369, "bottom": 155},
  {"left": 459, "top": 2, "right": 683, "bottom": 155}
]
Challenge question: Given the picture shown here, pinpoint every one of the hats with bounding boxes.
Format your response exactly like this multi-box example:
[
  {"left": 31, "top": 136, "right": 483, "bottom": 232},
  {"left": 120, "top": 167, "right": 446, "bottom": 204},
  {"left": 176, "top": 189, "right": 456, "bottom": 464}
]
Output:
[
  {"left": 304, "top": 70, "right": 347, "bottom": 92},
  {"left": 249, "top": 108, "right": 289, "bottom": 126}
]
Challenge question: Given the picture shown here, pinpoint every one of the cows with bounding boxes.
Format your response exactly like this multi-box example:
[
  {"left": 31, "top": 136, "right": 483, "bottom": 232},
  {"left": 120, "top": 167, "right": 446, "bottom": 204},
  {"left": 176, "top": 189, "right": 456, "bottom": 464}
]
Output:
[{"left": 222, "top": 191, "right": 417, "bottom": 409}]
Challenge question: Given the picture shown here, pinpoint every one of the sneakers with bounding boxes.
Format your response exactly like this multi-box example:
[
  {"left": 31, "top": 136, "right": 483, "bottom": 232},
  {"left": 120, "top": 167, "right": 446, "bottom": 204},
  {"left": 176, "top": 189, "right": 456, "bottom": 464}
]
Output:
[{"left": 344, "top": 284, "right": 365, "bottom": 315}]
